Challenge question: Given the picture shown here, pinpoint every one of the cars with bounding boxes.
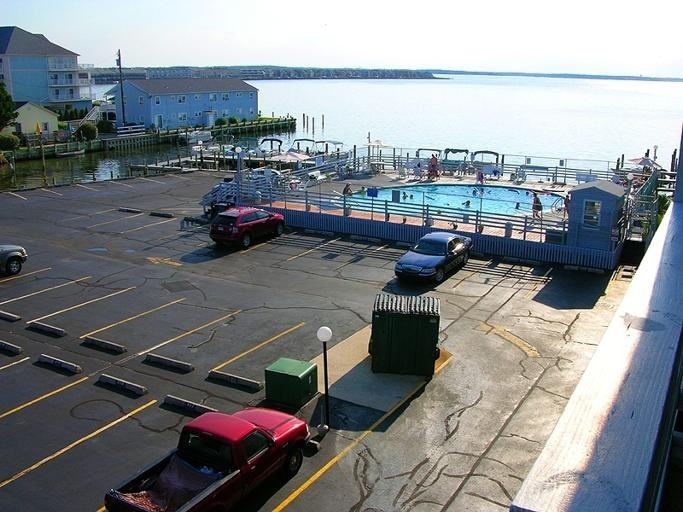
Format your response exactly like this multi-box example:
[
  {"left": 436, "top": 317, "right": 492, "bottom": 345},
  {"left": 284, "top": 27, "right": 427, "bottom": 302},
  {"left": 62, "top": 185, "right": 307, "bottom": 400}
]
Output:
[
  {"left": 394, "top": 231, "right": 474, "bottom": 284},
  {"left": 209, "top": 206, "right": 285, "bottom": 249},
  {"left": 0, "top": 244, "right": 29, "bottom": 276}
]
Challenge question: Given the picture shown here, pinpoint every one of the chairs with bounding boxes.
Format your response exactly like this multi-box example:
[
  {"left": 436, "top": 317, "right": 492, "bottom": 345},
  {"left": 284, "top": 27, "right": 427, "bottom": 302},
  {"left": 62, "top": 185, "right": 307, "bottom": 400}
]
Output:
[{"left": 332, "top": 190, "right": 358, "bottom": 197}]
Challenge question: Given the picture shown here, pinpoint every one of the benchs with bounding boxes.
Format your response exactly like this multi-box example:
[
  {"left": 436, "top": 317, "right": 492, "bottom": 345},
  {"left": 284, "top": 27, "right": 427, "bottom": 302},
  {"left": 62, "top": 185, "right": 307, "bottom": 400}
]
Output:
[{"left": 305, "top": 170, "right": 328, "bottom": 187}]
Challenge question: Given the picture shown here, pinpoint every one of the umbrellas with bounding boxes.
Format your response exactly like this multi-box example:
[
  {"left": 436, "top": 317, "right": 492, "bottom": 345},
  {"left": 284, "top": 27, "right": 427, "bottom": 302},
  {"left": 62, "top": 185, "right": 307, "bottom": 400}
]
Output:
[
  {"left": 628, "top": 154, "right": 662, "bottom": 173},
  {"left": 33, "top": 120, "right": 42, "bottom": 134}
]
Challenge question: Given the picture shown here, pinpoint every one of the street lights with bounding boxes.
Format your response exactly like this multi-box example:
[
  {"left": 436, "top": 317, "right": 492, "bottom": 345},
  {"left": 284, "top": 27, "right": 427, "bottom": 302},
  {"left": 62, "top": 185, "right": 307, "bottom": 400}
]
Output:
[
  {"left": 624, "top": 172, "right": 634, "bottom": 228},
  {"left": 316, "top": 325, "right": 333, "bottom": 432},
  {"left": 235, "top": 146, "right": 242, "bottom": 173},
  {"left": 198, "top": 140, "right": 203, "bottom": 162}
]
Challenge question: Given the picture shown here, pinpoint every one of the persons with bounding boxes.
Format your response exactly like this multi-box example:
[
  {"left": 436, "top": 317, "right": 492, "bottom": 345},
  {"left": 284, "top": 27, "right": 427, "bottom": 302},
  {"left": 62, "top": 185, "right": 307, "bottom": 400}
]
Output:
[
  {"left": 461, "top": 199, "right": 470, "bottom": 206},
  {"left": 532, "top": 192, "right": 541, "bottom": 223},
  {"left": 353, "top": 185, "right": 367, "bottom": 196},
  {"left": 401, "top": 191, "right": 408, "bottom": 200},
  {"left": 409, "top": 194, "right": 413, "bottom": 199},
  {"left": 470, "top": 164, "right": 531, "bottom": 199},
  {"left": 341, "top": 182, "right": 352, "bottom": 196},
  {"left": 428, "top": 153, "right": 439, "bottom": 178},
  {"left": 515, "top": 202, "right": 521, "bottom": 209},
  {"left": 563, "top": 194, "right": 569, "bottom": 218},
  {"left": 413, "top": 165, "right": 426, "bottom": 180}
]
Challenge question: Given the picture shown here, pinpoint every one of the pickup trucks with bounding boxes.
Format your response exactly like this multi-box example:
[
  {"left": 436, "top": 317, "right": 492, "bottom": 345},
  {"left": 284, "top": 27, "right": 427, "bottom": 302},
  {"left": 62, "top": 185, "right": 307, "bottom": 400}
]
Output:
[{"left": 104, "top": 405, "right": 311, "bottom": 512}]
